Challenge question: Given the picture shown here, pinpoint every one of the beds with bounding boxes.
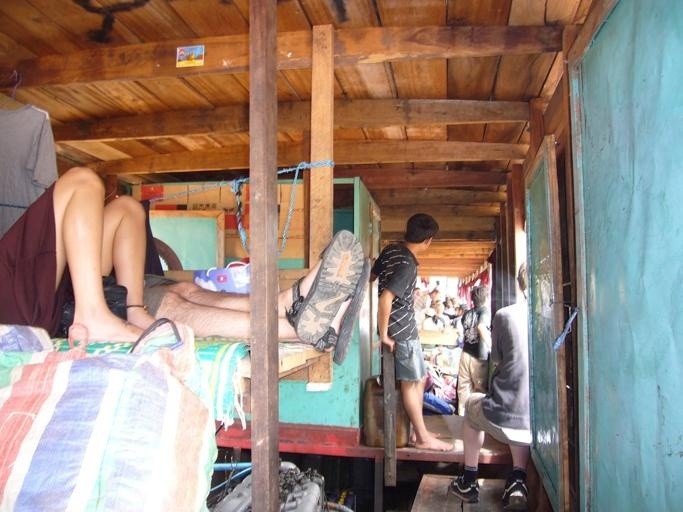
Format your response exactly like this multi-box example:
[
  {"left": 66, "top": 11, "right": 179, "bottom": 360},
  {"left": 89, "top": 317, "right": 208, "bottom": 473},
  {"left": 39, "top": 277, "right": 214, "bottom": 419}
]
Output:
[{"left": 1, "top": 313, "right": 336, "bottom": 392}]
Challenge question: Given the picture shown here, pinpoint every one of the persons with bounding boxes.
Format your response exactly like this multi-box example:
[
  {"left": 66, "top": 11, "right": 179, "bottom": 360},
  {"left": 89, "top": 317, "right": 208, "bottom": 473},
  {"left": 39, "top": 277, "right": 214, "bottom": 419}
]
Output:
[
  {"left": 447, "top": 260, "right": 531, "bottom": 511},
  {"left": 457, "top": 284, "right": 492, "bottom": 414},
  {"left": 369, "top": 213, "right": 453, "bottom": 451},
  {"left": 0, "top": 166, "right": 158, "bottom": 343},
  {"left": 109, "top": 229, "right": 373, "bottom": 366},
  {"left": 407, "top": 277, "right": 469, "bottom": 373}
]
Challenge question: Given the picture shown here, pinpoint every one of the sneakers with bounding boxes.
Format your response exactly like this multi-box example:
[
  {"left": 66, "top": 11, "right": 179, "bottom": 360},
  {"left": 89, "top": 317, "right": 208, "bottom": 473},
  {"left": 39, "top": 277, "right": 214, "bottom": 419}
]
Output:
[
  {"left": 500, "top": 474, "right": 529, "bottom": 511},
  {"left": 448, "top": 472, "right": 480, "bottom": 503}
]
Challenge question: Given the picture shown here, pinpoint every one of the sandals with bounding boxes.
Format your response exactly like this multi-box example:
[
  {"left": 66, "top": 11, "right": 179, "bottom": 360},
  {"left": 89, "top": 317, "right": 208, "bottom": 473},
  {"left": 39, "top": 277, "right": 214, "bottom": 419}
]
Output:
[
  {"left": 311, "top": 257, "right": 371, "bottom": 366},
  {"left": 287, "top": 229, "right": 364, "bottom": 344}
]
